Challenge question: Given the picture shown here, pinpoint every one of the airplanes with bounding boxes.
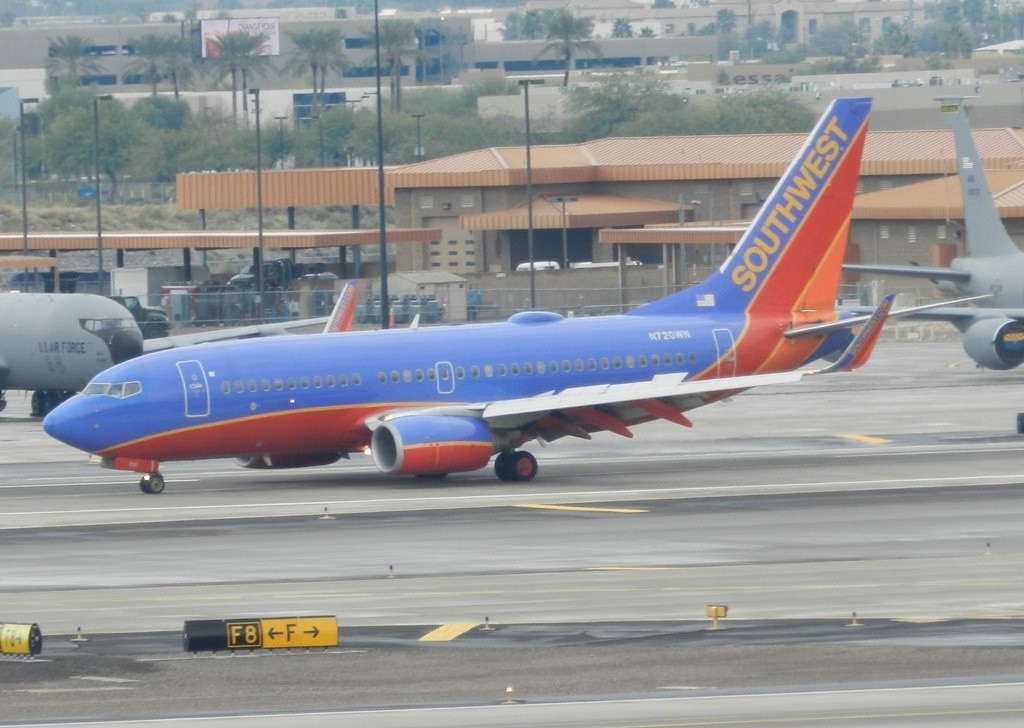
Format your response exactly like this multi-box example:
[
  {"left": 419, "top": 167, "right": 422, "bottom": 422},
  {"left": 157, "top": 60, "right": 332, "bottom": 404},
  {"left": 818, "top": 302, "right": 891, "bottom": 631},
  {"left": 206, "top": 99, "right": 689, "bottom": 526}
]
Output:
[
  {"left": 38, "top": 96, "right": 1001, "bottom": 495},
  {"left": 0, "top": 290, "right": 332, "bottom": 419},
  {"left": 831, "top": 94, "right": 1024, "bottom": 435}
]
